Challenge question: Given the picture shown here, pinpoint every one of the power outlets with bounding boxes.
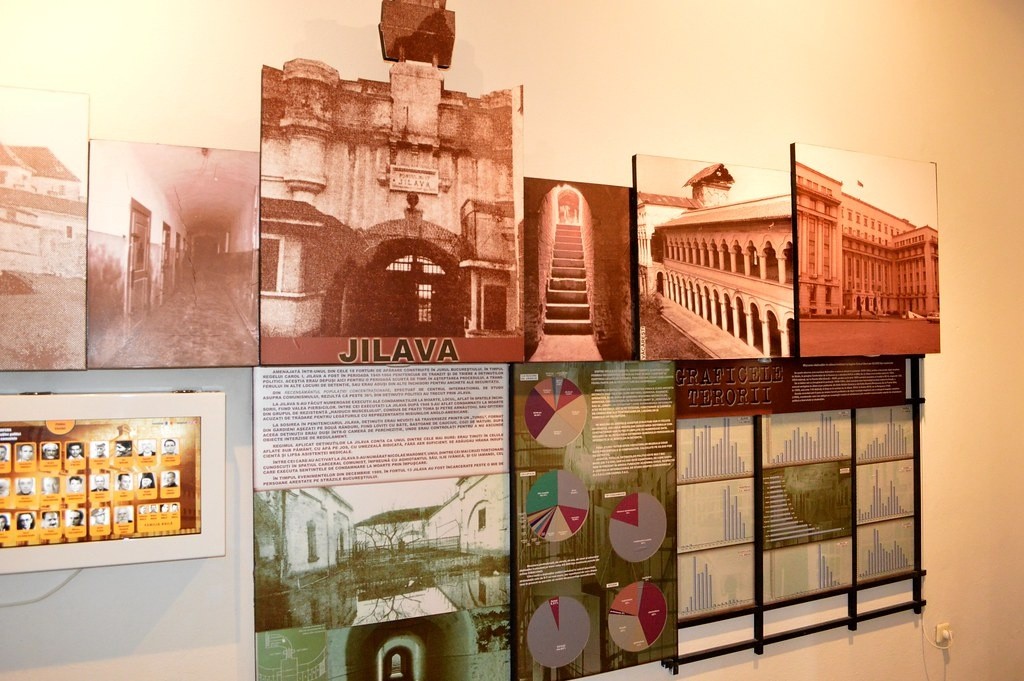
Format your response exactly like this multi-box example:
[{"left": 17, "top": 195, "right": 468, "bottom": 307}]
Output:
[{"left": 935, "top": 621, "right": 952, "bottom": 645}]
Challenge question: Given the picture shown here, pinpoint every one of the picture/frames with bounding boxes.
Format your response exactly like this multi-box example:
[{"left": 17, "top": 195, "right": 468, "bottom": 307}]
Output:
[{"left": 3, "top": 62, "right": 941, "bottom": 681}]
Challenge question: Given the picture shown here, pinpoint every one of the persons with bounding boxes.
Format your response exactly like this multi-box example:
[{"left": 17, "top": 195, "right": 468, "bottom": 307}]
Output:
[
  {"left": 0, "top": 445, "right": 9, "bottom": 463},
  {"left": 17, "top": 513, "right": 35, "bottom": 530},
  {"left": 16, "top": 478, "right": 34, "bottom": 495},
  {"left": 91, "top": 508, "right": 106, "bottom": 525},
  {"left": 69, "top": 475, "right": 83, "bottom": 493},
  {"left": 68, "top": 443, "right": 83, "bottom": 459},
  {"left": 68, "top": 510, "right": 84, "bottom": 527},
  {"left": 116, "top": 441, "right": 132, "bottom": 456},
  {"left": 117, "top": 474, "right": 131, "bottom": 490},
  {"left": 43, "top": 443, "right": 59, "bottom": 460},
  {"left": 162, "top": 440, "right": 178, "bottom": 456},
  {"left": 0, "top": 515, "right": 9, "bottom": 532},
  {"left": 43, "top": 477, "right": 59, "bottom": 494},
  {"left": 92, "top": 442, "right": 107, "bottom": 458},
  {"left": 139, "top": 440, "right": 155, "bottom": 456},
  {"left": 139, "top": 473, "right": 155, "bottom": 489},
  {"left": 139, "top": 504, "right": 179, "bottom": 515},
  {"left": 116, "top": 507, "right": 132, "bottom": 524},
  {"left": 0, "top": 478, "right": 10, "bottom": 495},
  {"left": 17, "top": 444, "right": 33, "bottom": 462},
  {"left": 163, "top": 472, "right": 178, "bottom": 487},
  {"left": 92, "top": 476, "right": 108, "bottom": 491},
  {"left": 42, "top": 511, "right": 60, "bottom": 528}
]
